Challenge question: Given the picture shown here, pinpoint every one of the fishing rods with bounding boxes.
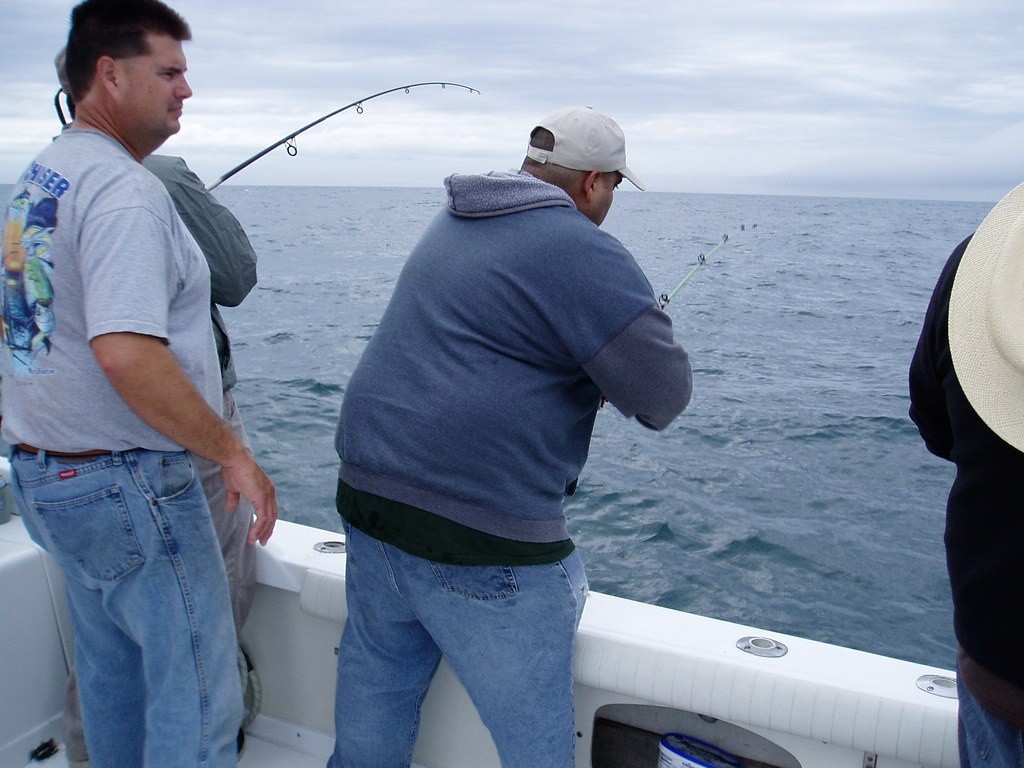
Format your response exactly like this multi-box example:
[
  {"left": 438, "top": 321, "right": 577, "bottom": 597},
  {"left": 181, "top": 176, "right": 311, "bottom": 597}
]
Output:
[
  {"left": 597, "top": 224, "right": 759, "bottom": 410},
  {"left": 204, "top": 82, "right": 481, "bottom": 192}
]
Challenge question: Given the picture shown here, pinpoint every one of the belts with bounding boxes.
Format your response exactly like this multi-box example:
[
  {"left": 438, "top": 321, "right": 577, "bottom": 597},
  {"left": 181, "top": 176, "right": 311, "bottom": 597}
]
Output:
[{"left": 17, "top": 440, "right": 111, "bottom": 458}]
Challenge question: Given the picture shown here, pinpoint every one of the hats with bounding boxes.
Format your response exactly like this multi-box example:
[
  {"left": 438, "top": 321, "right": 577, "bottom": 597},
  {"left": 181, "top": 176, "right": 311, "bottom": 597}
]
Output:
[
  {"left": 948, "top": 183, "right": 1024, "bottom": 450},
  {"left": 528, "top": 106, "right": 644, "bottom": 191}
]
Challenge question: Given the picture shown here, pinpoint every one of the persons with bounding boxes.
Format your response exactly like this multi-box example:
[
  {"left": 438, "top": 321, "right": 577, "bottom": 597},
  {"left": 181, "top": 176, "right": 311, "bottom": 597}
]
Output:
[
  {"left": 908, "top": 182, "right": 1024, "bottom": 768},
  {"left": 0, "top": 0, "right": 278, "bottom": 768},
  {"left": 327, "top": 105, "right": 693, "bottom": 768}
]
